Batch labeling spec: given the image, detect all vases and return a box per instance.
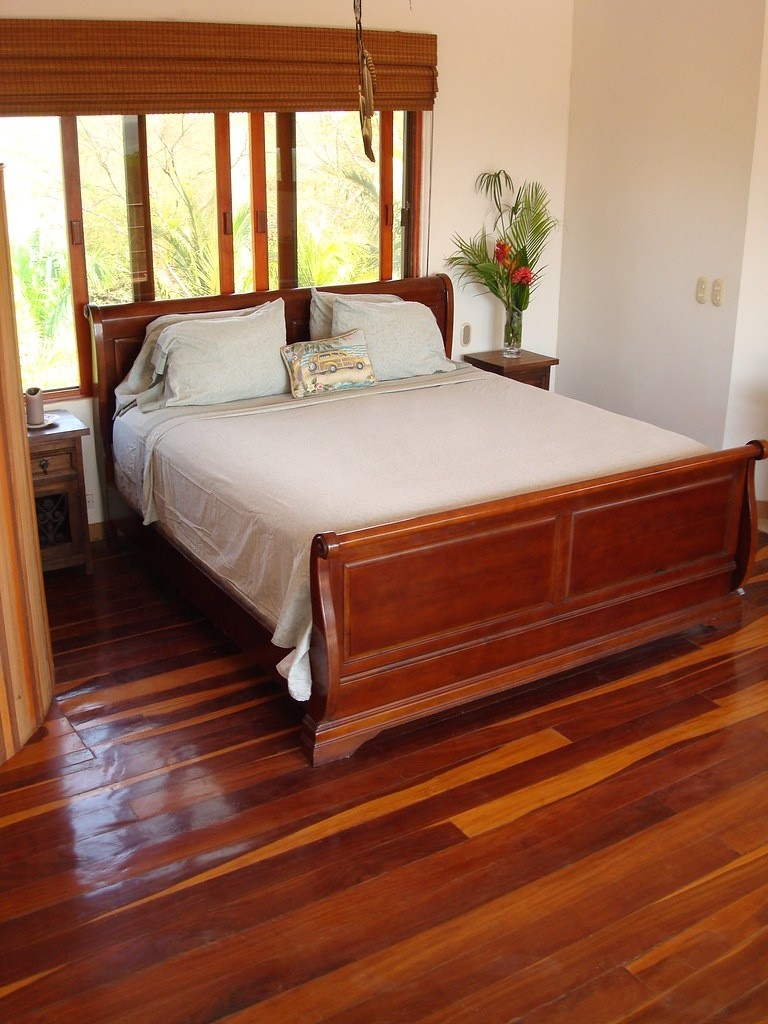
[502,304,525,359]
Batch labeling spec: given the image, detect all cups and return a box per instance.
[26,387,44,424]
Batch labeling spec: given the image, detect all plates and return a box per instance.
[25,413,60,429]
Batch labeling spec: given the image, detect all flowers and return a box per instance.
[443,167,561,347]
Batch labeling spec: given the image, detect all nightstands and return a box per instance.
[25,406,92,576]
[461,346,559,391]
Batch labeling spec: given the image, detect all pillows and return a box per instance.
[330,297,471,380]
[280,327,377,401]
[147,296,292,409]
[309,287,404,339]
[113,302,269,410]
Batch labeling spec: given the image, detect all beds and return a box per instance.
[83,273,767,772]
